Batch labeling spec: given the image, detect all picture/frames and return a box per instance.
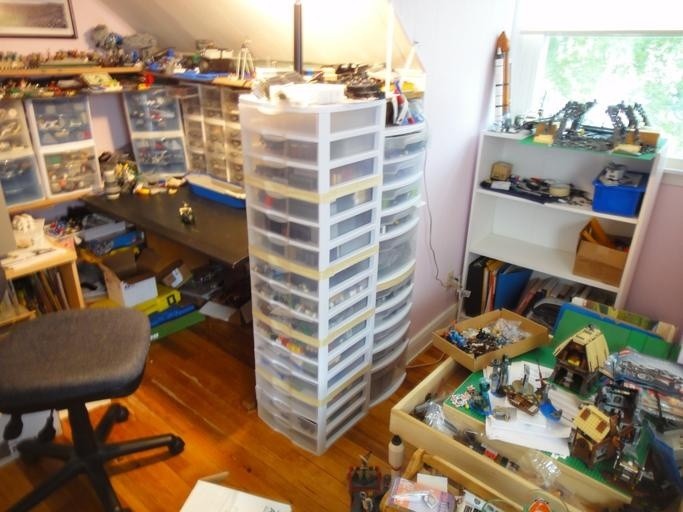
[0,0,78,39]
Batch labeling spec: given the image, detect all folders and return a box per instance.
[494,264,533,309]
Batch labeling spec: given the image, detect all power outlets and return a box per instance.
[451,273,461,286]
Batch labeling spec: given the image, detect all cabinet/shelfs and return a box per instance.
[24,93,104,200]
[176,80,245,190]
[0,97,46,207]
[122,83,189,178]
[455,124,671,322]
[0,225,86,308]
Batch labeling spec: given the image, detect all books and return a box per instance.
[7,267,71,315]
[513,277,612,318]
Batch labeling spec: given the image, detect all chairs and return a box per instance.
[0,264,184,511]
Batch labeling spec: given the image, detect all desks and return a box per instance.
[79,183,249,272]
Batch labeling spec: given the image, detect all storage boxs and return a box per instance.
[0,310,64,466]
[197,289,253,328]
[95,246,159,308]
[591,168,649,218]
[205,317,254,369]
[159,258,194,289]
[179,270,227,310]
[572,218,632,288]
